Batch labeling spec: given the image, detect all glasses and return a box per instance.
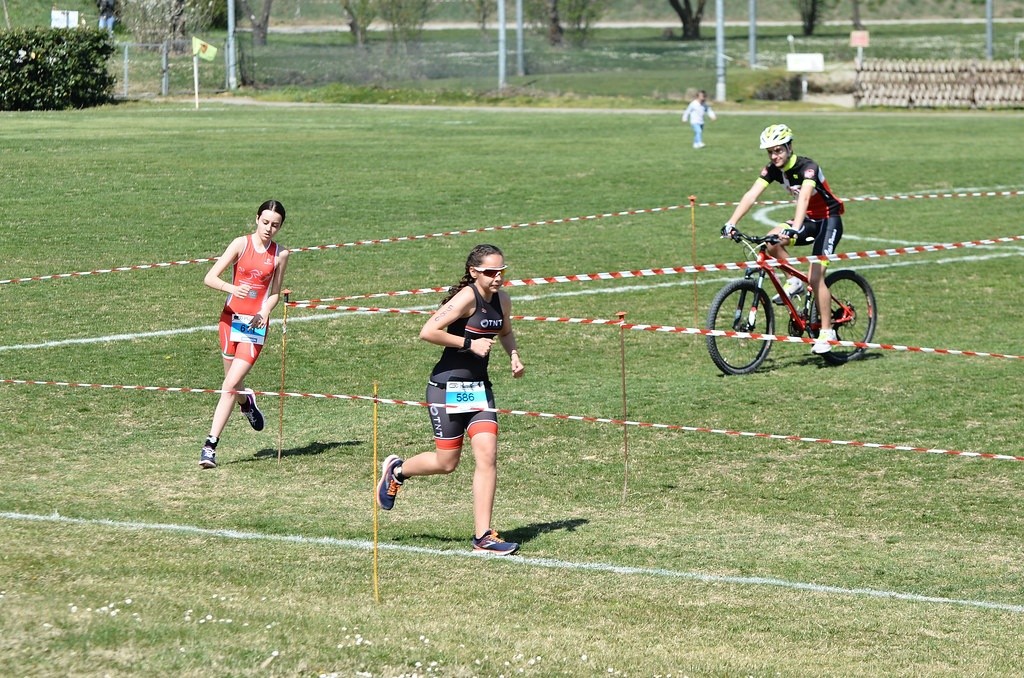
[473,265,508,278]
[768,146,786,153]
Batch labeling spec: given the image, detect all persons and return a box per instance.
[96,0,118,38]
[721,124,844,354]
[198,200,290,468]
[377,244,524,555]
[681,90,717,148]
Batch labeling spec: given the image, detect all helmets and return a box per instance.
[759,124,792,149]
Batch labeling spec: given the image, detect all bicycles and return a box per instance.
[702,229,881,373]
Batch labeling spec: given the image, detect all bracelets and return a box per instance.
[510,352,520,360]
[458,338,471,352]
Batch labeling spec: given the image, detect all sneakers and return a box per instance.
[240,388,264,431]
[811,329,838,353]
[377,455,404,510]
[772,278,804,305]
[199,446,216,467]
[473,529,520,556]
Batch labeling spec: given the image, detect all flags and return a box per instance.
[193,37,218,61]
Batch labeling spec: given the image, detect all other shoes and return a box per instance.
[693,142,705,149]
